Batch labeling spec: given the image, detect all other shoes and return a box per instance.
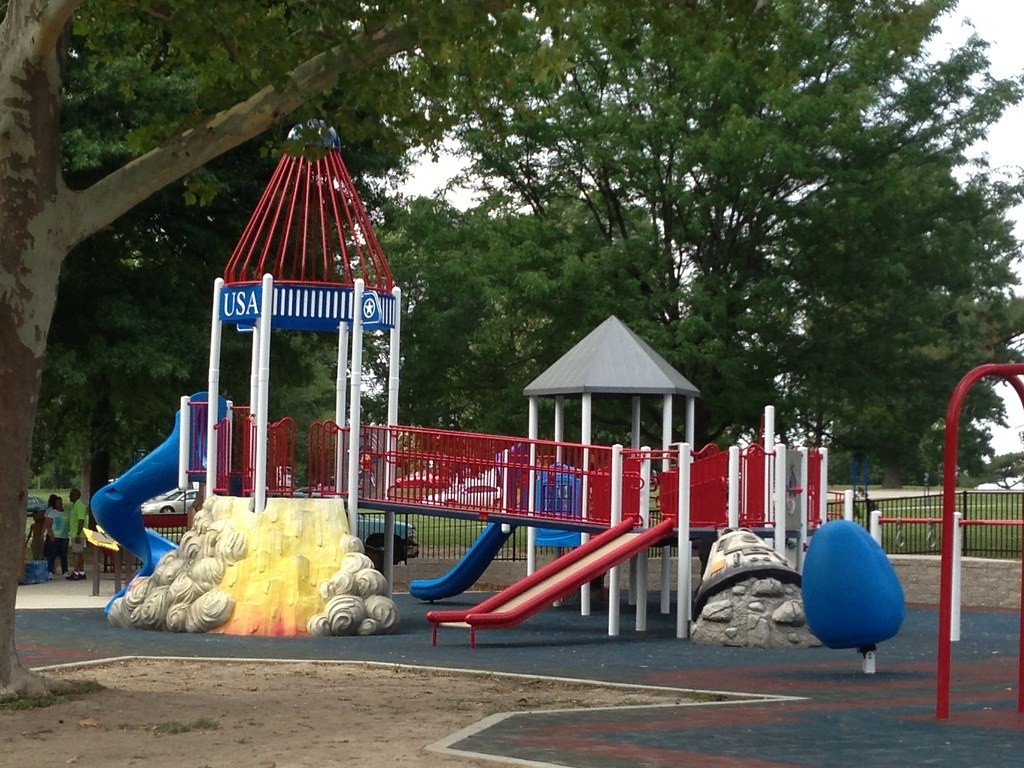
[49,572,54,580]
[63,572,70,577]
[65,572,86,581]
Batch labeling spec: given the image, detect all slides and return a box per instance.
[408,522,518,600]
[426,516,674,630]
[90,392,228,615]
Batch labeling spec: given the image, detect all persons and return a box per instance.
[923,473,932,494]
[25,494,70,580]
[65,489,87,581]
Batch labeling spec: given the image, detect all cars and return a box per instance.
[141,490,200,514]
[345,509,420,573]
